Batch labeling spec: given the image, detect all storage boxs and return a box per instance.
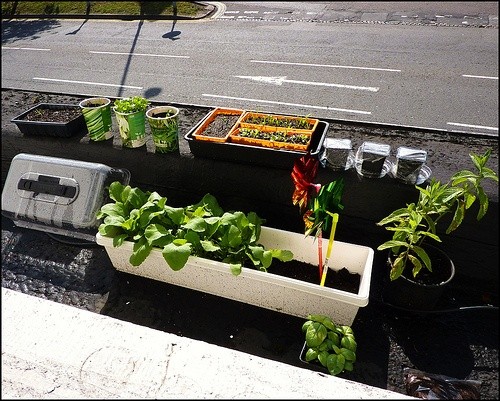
[1,152,132,242]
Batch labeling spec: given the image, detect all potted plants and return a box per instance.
[238,111,319,133]
[192,105,247,141]
[299,313,357,379]
[375,147,499,318]
[146,105,181,155]
[10,102,88,138]
[95,179,375,327]
[79,95,114,141]
[113,94,151,147]
[229,125,312,151]
